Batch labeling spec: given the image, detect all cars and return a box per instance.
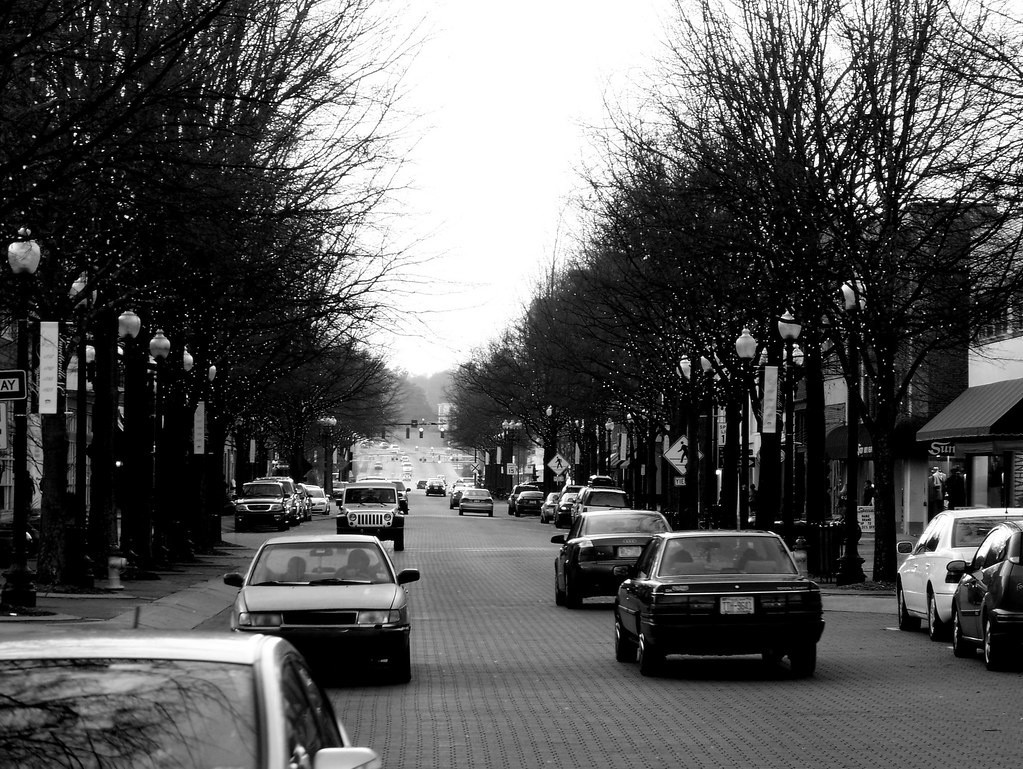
[946,520,1023,673]
[0,629,383,769]
[222,534,420,683]
[550,509,675,608]
[611,528,824,679]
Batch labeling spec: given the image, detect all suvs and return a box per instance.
[897,505,1023,643]
[228,437,630,552]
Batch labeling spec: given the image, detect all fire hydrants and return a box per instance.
[105,547,129,592]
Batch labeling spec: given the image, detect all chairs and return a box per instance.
[669,562,707,575]
[745,560,779,574]
[279,556,310,581]
[333,550,377,580]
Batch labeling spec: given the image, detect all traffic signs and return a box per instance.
[1,368,29,403]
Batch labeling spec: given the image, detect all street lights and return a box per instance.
[115,306,140,560]
[148,326,172,566]
[732,326,758,530]
[674,352,698,530]
[68,267,98,569]
[699,346,720,530]
[776,304,802,552]
[833,268,866,585]
[605,417,614,475]
[0,227,38,608]
[626,411,637,509]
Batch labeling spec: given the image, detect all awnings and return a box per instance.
[605,453,636,469]
[916,379,1023,440]
[826,420,911,458]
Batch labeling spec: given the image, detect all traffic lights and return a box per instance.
[440,427,444,438]
[419,427,424,438]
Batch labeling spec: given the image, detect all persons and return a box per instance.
[749,484,758,515]
[929,467,966,510]
[861,480,873,525]
[835,484,847,517]
[669,549,693,570]
[738,548,758,570]
[342,550,375,581]
[288,557,306,582]
